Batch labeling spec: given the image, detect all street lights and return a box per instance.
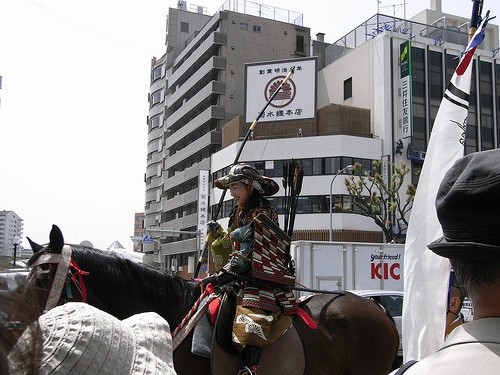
[329,164,353,241]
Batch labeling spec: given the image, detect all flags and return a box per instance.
[398,17,490,367]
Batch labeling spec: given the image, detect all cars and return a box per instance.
[346,287,416,363]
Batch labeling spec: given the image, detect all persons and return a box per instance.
[197,163,280,374]
[385,147,500,375]
[7,300,179,375]
[442,271,468,343]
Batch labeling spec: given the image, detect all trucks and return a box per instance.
[290,238,407,305]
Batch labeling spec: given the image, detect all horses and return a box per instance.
[10,223,401,375]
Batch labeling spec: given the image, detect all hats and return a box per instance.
[427,148,500,261]
[7,301,177,375]
[214,163,279,196]
